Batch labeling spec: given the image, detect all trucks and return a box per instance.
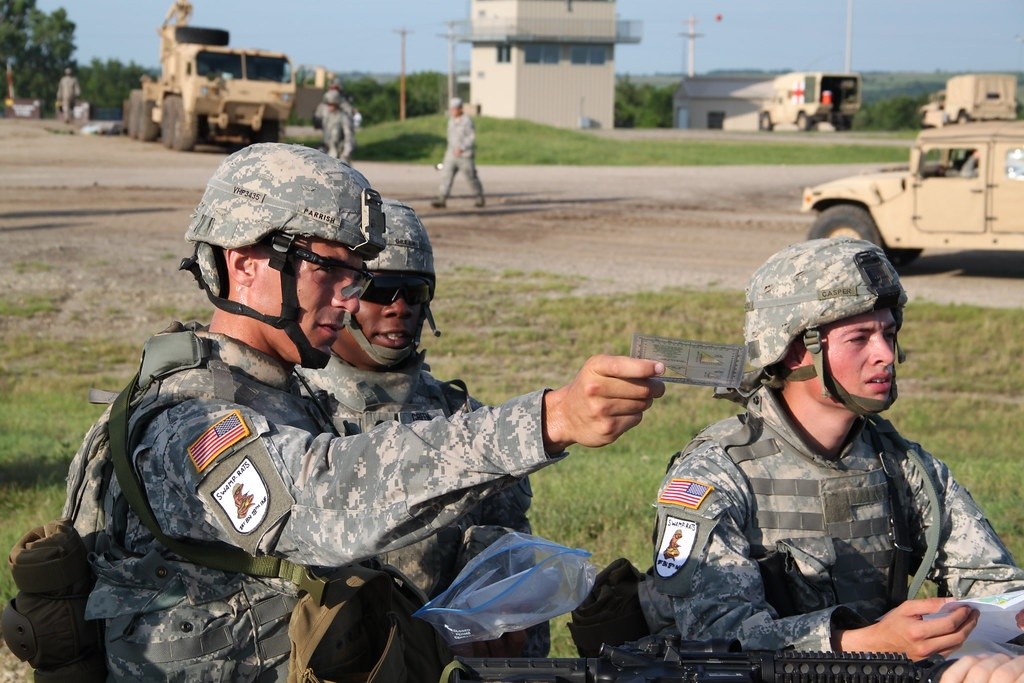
[920,73,1017,129]
[758,72,861,130]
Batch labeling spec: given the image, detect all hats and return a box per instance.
[450,97,462,107]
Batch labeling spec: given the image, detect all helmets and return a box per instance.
[183,141,387,298]
[742,233,908,369]
[362,199,435,278]
[321,89,341,105]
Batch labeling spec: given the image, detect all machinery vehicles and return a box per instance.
[293,66,332,127]
[122,1,296,152]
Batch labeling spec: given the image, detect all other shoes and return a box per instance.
[474,197,485,207]
[430,201,447,208]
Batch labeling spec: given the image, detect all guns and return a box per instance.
[449,632,960,683]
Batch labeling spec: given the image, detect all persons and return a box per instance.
[322,90,354,162]
[64,142,666,683]
[432,98,484,206]
[293,198,550,660]
[938,149,979,177]
[57,68,81,123]
[654,237,1023,683]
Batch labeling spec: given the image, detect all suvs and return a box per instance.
[798,120,1024,266]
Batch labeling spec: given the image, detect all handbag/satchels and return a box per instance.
[286,564,468,683]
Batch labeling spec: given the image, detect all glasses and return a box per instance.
[356,273,434,306]
